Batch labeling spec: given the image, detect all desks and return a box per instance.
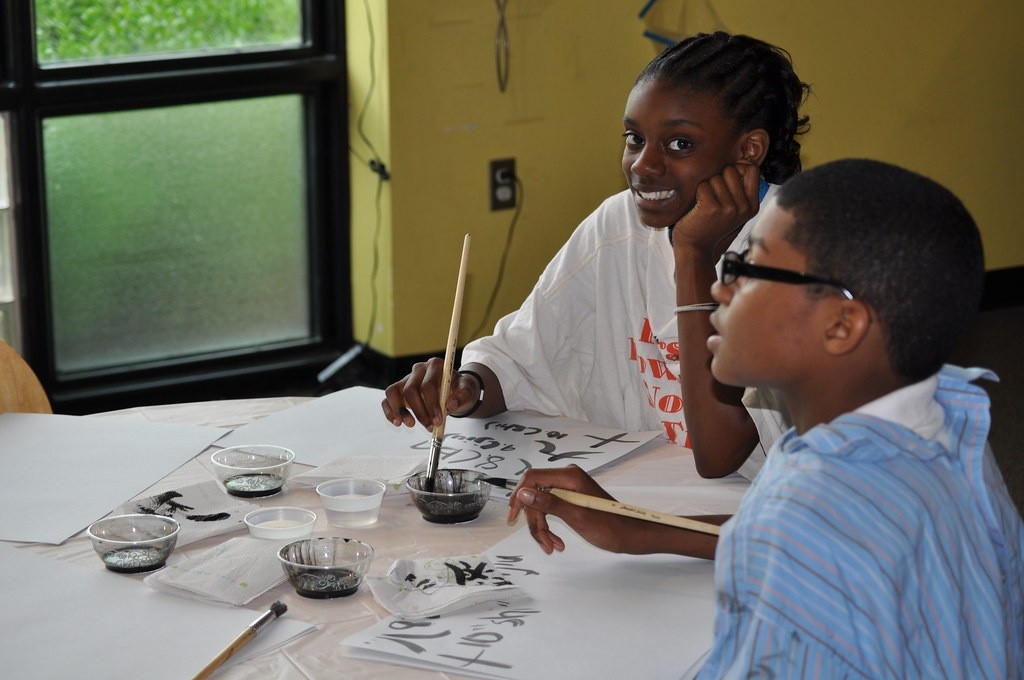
[0,396,751,680]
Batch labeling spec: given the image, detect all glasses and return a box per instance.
[716,251,876,324]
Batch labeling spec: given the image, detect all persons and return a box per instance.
[506,157,1023,680]
[380,31,812,480]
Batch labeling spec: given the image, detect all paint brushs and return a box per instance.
[423,234,473,493]
[503,476,721,541]
[191,601,288,680]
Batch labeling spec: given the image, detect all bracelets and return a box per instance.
[449,370,485,418]
[673,302,720,314]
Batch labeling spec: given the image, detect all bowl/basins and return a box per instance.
[210,444,296,498]
[86,514,182,574]
[315,477,387,528]
[405,468,493,526]
[276,536,375,599]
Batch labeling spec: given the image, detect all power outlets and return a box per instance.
[489,158,512,213]
